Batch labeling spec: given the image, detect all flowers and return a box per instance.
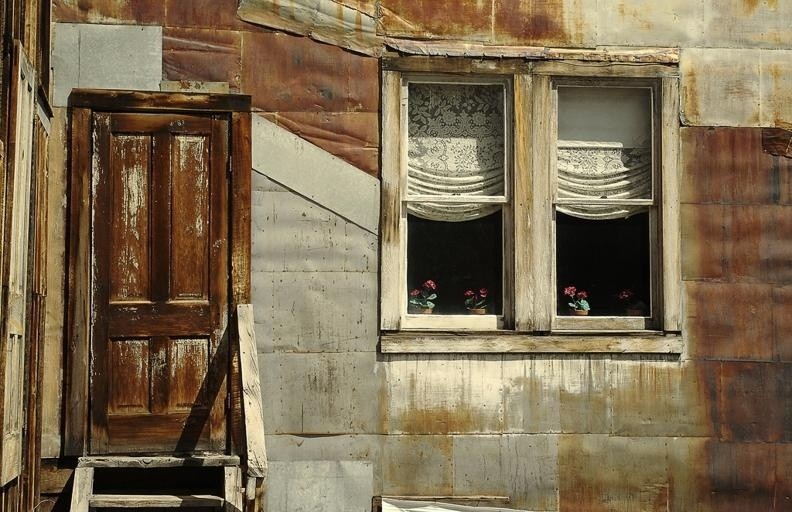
[463,287,489,309]
[563,283,591,311]
[408,279,437,309]
[615,287,649,310]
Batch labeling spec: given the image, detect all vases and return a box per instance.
[468,307,486,315]
[569,309,586,316]
[626,308,643,316]
[414,306,433,314]
